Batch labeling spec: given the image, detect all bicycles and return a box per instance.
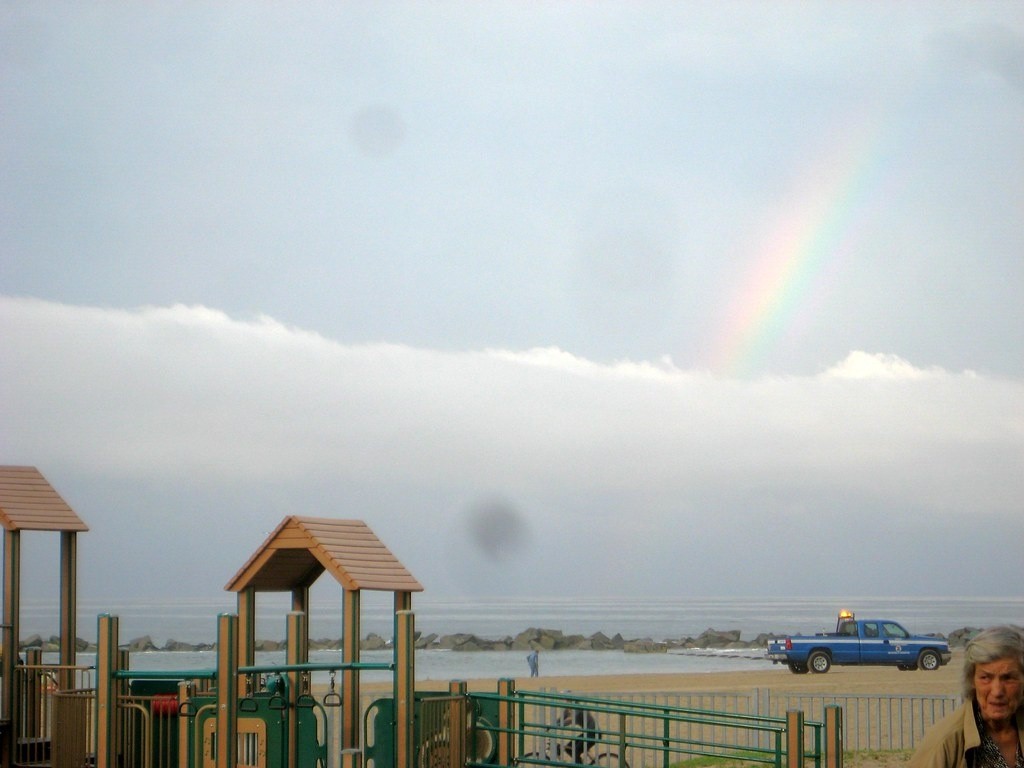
[516,728,630,768]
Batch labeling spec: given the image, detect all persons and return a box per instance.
[544,689,602,765]
[906,627,1024,768]
[526,650,539,678]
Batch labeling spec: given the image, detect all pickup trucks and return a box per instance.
[763,609,952,675]
